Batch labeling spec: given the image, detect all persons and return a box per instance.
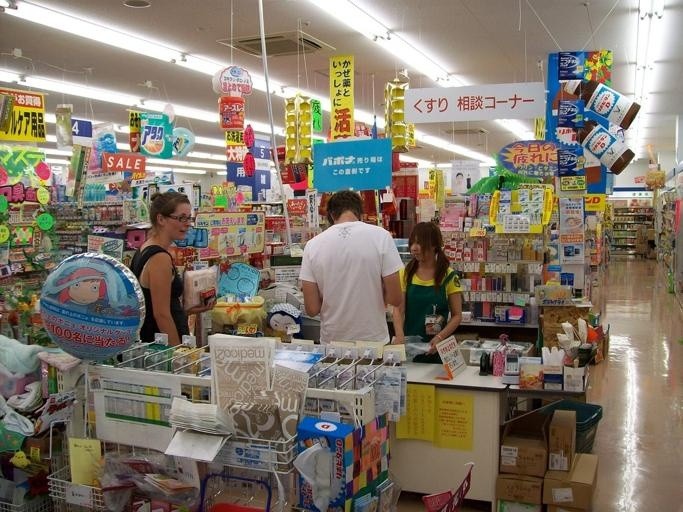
[129,190,196,348]
[391,220,465,363]
[453,173,467,194]
[296,190,405,365]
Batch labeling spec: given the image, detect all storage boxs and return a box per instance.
[494,410,599,512]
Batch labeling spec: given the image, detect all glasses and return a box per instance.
[169,215,192,223]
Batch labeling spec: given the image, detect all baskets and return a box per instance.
[545,400,602,453]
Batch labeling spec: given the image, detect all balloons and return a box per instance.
[38,252,146,364]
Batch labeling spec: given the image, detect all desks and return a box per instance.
[386,361,590,512]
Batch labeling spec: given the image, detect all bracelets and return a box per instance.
[436,334,444,341]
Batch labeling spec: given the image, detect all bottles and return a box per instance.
[81,182,122,222]
